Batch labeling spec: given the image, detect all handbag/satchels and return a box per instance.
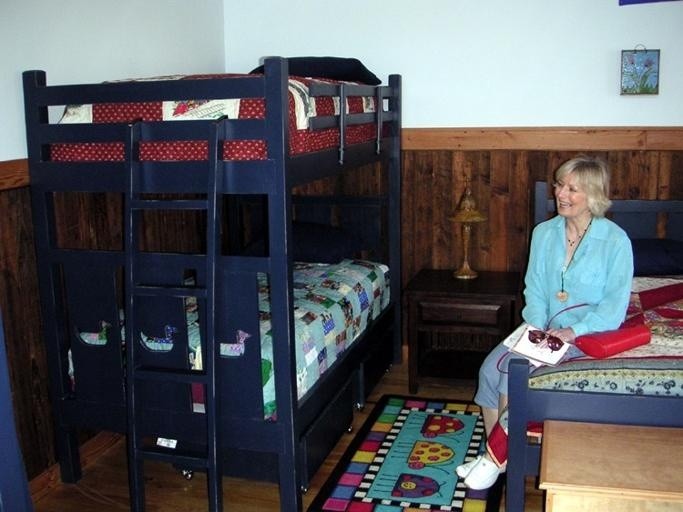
[571,323,652,360]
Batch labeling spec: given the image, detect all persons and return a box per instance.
[452,152,634,492]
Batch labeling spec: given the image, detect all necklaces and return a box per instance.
[563,222,590,246]
[556,215,593,303]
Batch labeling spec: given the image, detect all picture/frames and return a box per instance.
[620,49,660,95]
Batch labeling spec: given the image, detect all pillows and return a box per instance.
[250,56,381,85]
[631,239,683,276]
[242,221,354,264]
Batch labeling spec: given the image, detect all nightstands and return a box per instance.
[408,269,524,395]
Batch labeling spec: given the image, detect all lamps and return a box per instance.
[451,186,489,283]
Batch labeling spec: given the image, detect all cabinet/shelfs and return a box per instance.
[539,419,683,512]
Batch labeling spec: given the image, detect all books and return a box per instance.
[503,322,572,369]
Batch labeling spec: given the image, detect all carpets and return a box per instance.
[306,393,504,512]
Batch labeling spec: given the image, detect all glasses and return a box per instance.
[528,329,565,352]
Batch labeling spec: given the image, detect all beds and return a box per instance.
[508,180,683,512]
[21,57,404,508]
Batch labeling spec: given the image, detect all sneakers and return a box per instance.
[455,453,507,480]
[462,454,501,492]
